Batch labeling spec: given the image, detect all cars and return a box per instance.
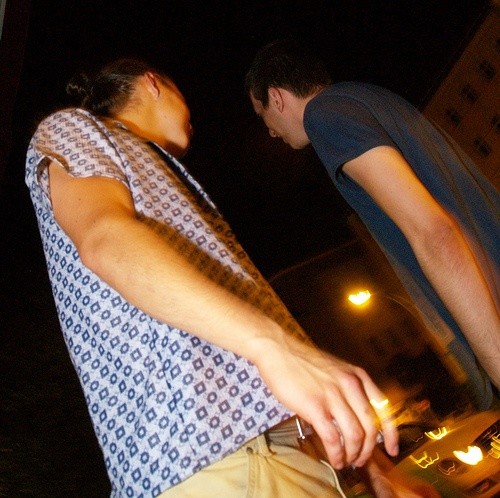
[340,410,499,498]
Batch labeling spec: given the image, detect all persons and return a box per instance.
[25,58,442,498]
[247,55,500,408]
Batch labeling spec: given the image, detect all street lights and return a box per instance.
[349,290,462,376]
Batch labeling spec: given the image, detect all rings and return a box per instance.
[373,398,391,409]
